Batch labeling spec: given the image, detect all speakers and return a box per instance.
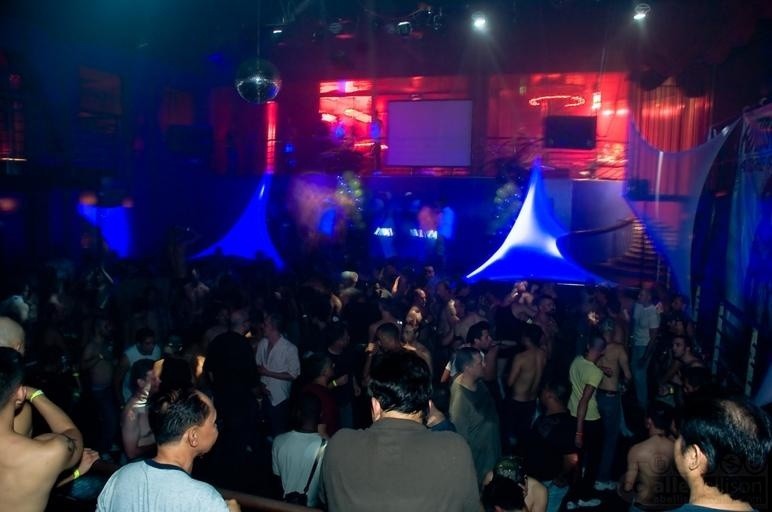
[544,116,596,149]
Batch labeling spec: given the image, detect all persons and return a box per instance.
[319,347,481,512]
[673,385,770,512]
[374,266,711,511]
[2,261,159,511]
[158,262,382,511]
[97,380,240,511]
[1,347,83,511]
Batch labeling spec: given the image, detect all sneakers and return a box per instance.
[566,496,604,511]
[592,479,619,493]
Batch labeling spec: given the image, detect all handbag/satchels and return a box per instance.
[283,437,327,508]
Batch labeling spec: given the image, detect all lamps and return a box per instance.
[232,0,282,106]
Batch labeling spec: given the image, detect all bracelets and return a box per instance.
[30,390,44,402]
[72,466,79,479]
